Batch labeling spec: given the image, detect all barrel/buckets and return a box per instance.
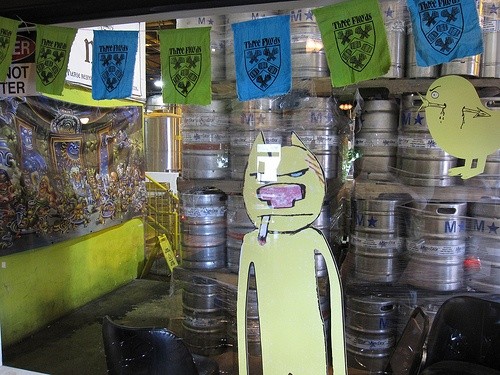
[179,0,500,372]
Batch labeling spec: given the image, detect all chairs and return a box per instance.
[421,297,500,375]
[378,305,430,375]
[102,316,221,375]
